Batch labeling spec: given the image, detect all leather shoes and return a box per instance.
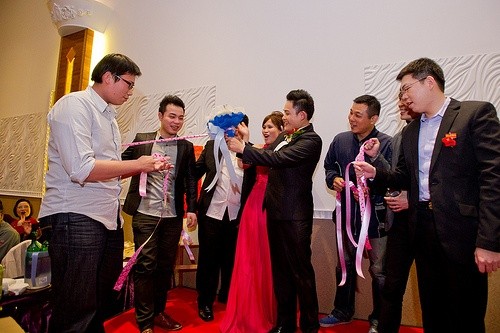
[198,304,214,321]
[141,311,183,333]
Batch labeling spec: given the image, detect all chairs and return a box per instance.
[1,240,45,280]
[175,218,200,286]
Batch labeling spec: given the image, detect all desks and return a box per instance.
[0,257,131,333]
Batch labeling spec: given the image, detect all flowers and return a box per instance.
[442,132,457,148]
[284,127,306,144]
[204,102,249,195]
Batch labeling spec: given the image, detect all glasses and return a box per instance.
[112,73,135,90]
[400,76,428,94]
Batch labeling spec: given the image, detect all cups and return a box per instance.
[0,262,3,296]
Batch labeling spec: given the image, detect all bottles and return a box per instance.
[26,232,41,278]
[385,187,401,197]
[40,241,51,274]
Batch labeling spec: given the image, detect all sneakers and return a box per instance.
[369,318,379,332]
[320,313,354,327]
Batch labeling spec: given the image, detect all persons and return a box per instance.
[319,96,391,328]
[122,95,198,333]
[195,89,320,333]
[396,58,500,333]
[10,200,42,238]
[0,200,21,265]
[38,53,175,333]
[370,87,422,333]
